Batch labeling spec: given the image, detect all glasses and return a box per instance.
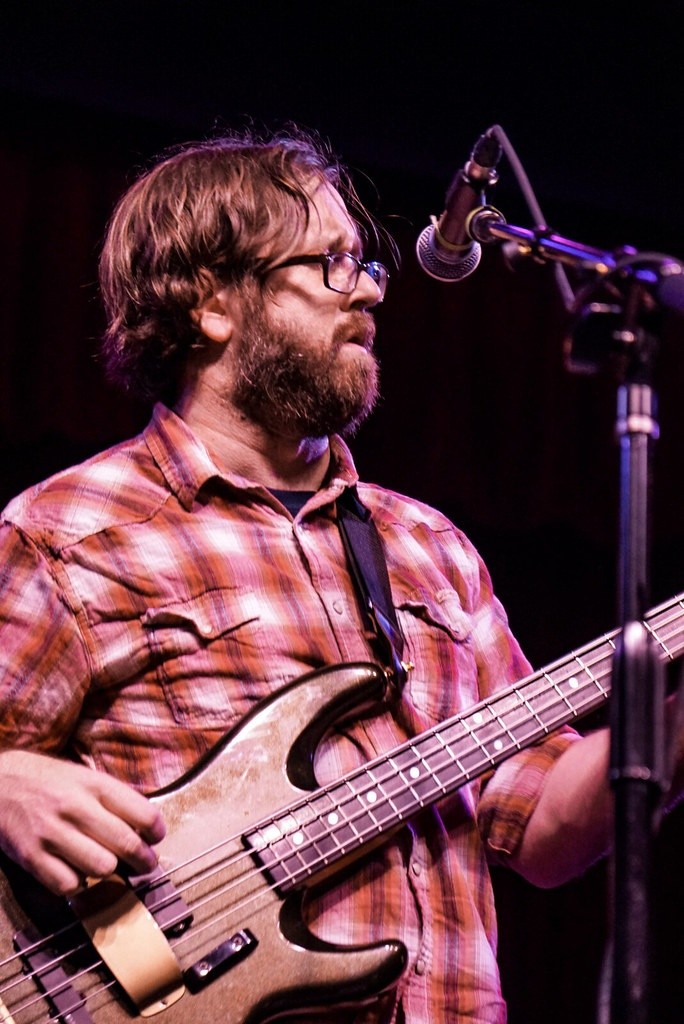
[258,252,391,304]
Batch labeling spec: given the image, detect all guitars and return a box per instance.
[1,589,684,1024]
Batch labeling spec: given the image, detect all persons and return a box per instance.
[0,140,684,1024]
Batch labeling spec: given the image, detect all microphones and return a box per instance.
[417,132,504,282]
